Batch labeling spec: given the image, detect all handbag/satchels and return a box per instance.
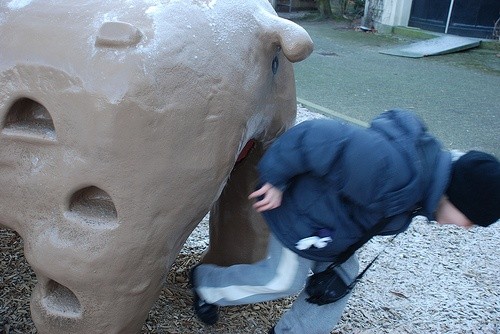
[304,266,351,308]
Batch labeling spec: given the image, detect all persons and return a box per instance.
[189,109,500,334]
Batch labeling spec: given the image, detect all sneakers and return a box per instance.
[188,263,220,326]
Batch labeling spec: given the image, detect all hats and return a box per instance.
[446,146,500,228]
